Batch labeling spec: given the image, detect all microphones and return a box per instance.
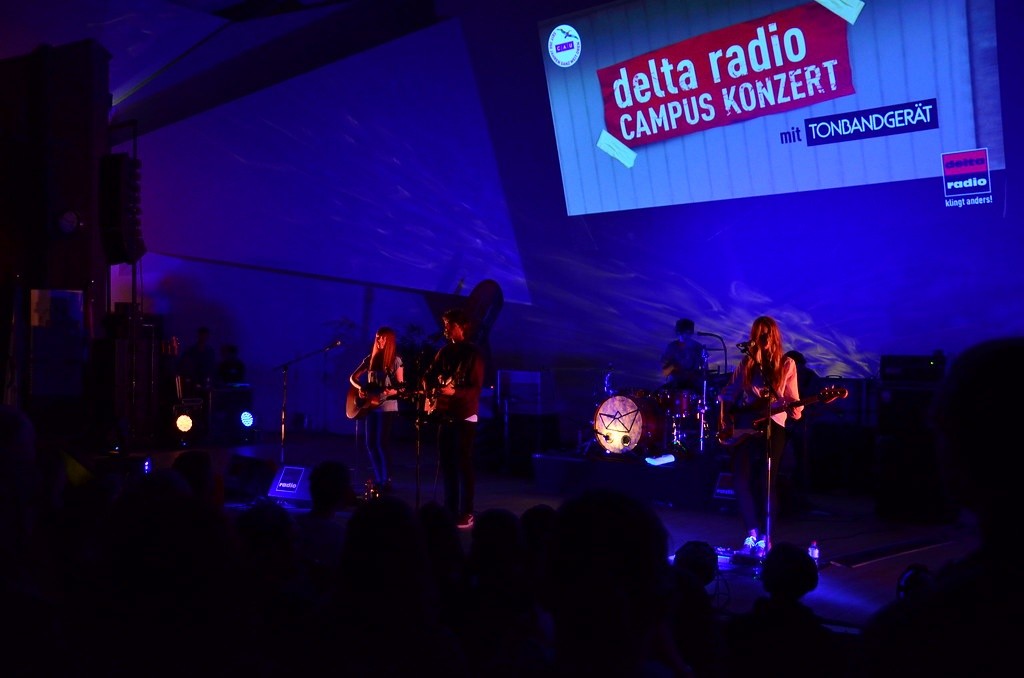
[697,332,711,336]
[325,340,342,352]
[737,340,755,347]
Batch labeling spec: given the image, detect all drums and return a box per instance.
[590,373,732,455]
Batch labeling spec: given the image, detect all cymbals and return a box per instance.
[697,369,718,374]
[693,347,724,351]
[594,368,627,374]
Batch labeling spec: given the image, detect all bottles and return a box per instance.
[808,540,819,569]
[364,479,374,499]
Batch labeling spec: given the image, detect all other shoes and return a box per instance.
[755,534,772,557]
[455,512,475,529]
[739,535,757,555]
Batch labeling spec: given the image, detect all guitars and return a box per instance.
[346,362,464,421]
[716,383,849,447]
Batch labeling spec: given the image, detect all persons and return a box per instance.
[0,340,1024,678]
[85,312,134,433]
[421,308,485,528]
[718,316,805,556]
[347,328,404,486]
[176,326,245,438]
[662,318,709,385]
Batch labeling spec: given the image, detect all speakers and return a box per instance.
[99,151,148,266]
[268,464,314,510]
[97,454,153,501]
[482,411,560,476]
[809,423,878,497]
